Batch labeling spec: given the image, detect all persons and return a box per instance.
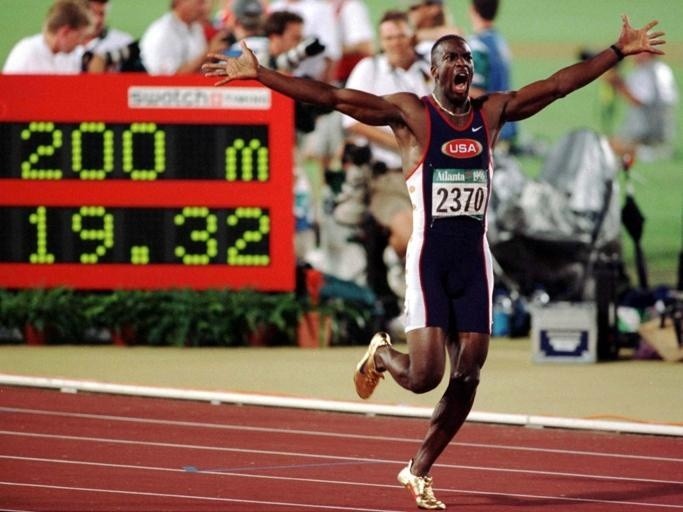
[272,2,513,315]
[606,36,666,161]
[201,13,667,511]
[6,1,301,76]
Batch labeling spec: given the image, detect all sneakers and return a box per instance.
[396,458,446,510]
[353,332,392,399]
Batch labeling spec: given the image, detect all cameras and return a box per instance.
[81,40,144,73]
[269,37,325,71]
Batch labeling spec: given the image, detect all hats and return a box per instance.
[230,0,262,26]
